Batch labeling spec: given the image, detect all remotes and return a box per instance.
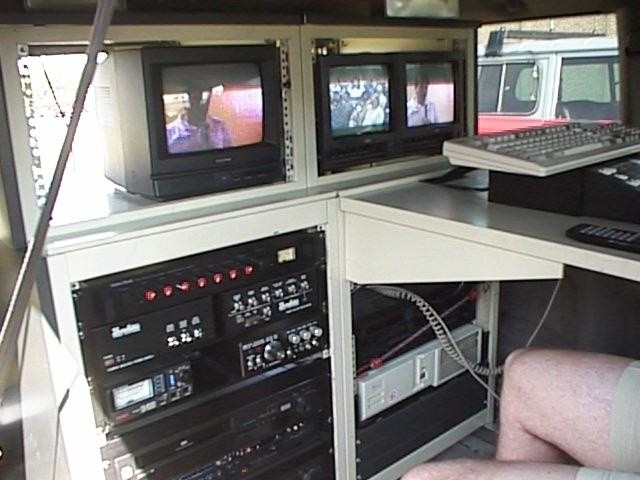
[565,223,639,253]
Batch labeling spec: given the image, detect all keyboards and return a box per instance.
[443,121,639,177]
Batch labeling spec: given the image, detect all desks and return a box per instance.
[327,167,640,480]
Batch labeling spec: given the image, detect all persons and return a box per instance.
[399,347,640,480]
[331,74,389,133]
[165,86,235,154]
[407,72,439,130]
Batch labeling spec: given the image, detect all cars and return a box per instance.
[477,26,619,133]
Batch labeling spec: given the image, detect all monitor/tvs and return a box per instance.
[312,52,467,177]
[94,41,286,203]
